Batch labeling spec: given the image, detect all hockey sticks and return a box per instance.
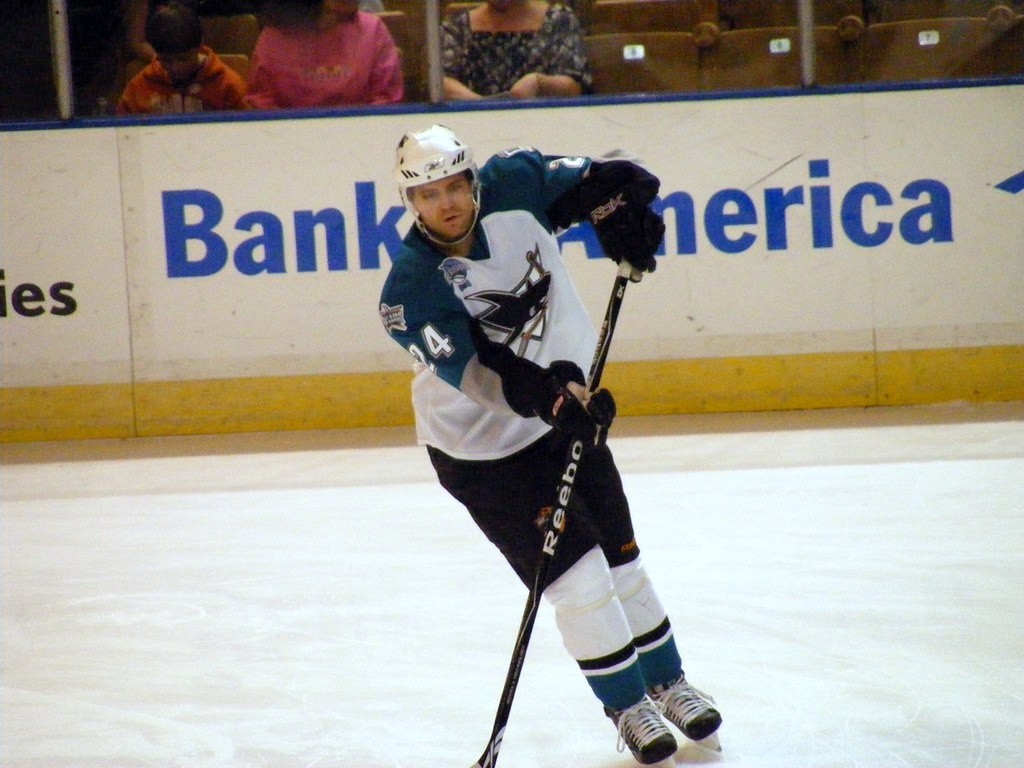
[472,253,636,768]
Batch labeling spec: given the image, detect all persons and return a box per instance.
[377,124,723,768]
[110,0,248,116]
[242,0,406,110]
[440,0,592,100]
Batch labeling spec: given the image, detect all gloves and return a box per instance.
[532,360,616,446]
[586,171,665,272]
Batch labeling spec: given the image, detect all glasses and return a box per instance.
[155,46,196,63]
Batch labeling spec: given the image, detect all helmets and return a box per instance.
[392,127,481,217]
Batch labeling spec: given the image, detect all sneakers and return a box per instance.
[647,671,723,752]
[604,694,678,768]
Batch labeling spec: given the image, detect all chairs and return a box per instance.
[124,0,1024,106]
